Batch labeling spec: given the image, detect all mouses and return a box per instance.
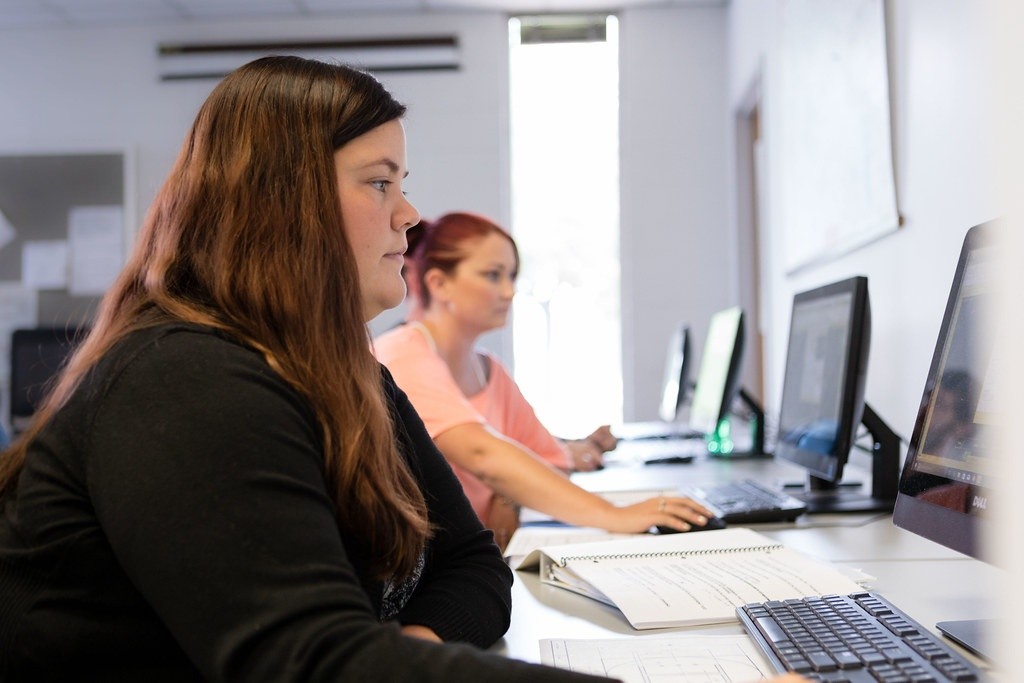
[657,516,726,535]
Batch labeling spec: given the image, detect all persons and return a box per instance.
[363,219,617,472]
[369,214,714,550]
[0,56,815,683]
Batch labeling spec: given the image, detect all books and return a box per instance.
[513,528,875,630]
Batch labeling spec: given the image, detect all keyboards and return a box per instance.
[683,477,807,525]
[735,591,1003,683]
[643,455,694,464]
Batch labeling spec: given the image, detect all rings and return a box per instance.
[660,501,665,513]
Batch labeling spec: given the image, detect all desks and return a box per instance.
[476,418,1000,683]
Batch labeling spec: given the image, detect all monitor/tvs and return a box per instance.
[657,328,706,441]
[892,211,1017,662]
[688,307,774,460]
[13,327,91,432]
[773,276,903,515]
